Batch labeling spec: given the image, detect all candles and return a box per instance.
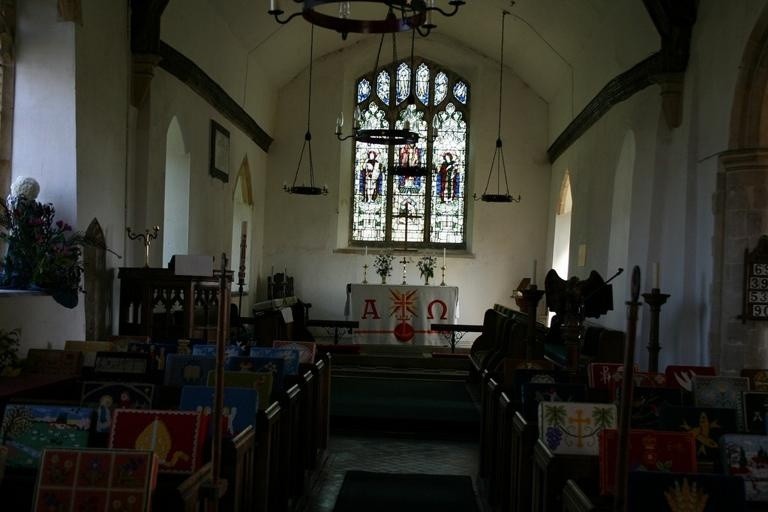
[364,244,367,267]
[649,257,660,289]
[282,263,287,281]
[269,263,274,283]
[442,246,446,267]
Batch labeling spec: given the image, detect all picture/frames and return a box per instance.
[206,118,235,184]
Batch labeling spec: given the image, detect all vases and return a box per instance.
[423,271,429,285]
[380,272,386,285]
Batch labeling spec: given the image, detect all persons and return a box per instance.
[358,151,386,202]
[438,153,460,203]
[399,143,422,188]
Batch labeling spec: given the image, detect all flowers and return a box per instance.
[414,254,438,279]
[0,171,87,311]
[372,243,396,276]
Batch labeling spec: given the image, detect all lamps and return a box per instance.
[281,18,333,197]
[471,9,524,203]
[334,4,439,147]
[264,0,465,39]
[381,141,438,181]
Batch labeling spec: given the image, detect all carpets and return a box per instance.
[329,468,478,511]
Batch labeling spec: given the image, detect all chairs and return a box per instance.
[478,365,766,512]
[0,333,333,512]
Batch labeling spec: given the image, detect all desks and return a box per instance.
[342,282,458,354]
